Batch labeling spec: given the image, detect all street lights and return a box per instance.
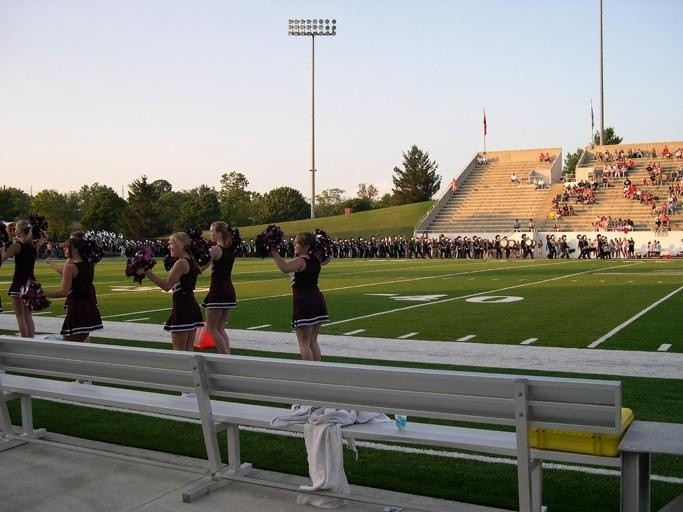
[286,17,335,218]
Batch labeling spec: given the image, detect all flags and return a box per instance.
[483,113,486,136]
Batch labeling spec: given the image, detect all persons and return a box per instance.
[37,238,104,342]
[134,231,204,352]
[415,145,683,261]
[190,220,237,354]
[269,231,332,361]
[0,220,38,339]
[39,228,415,260]
[45,231,99,342]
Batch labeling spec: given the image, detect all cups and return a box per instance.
[393,414,408,432]
